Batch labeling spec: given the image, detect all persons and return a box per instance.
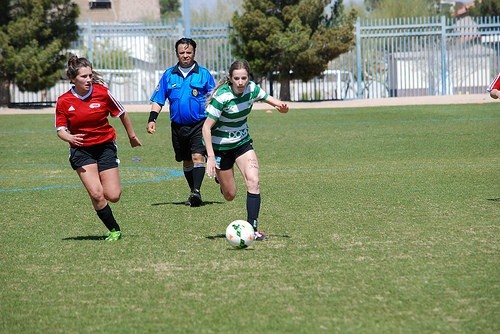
[146,38,216,207]
[54,56,142,241]
[486,73,500,99]
[203,59,289,240]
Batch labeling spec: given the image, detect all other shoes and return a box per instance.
[185,188,204,207]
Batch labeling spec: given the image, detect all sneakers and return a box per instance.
[105,228,121,242]
[253,231,268,241]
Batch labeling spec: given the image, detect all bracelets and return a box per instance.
[148,111,159,124]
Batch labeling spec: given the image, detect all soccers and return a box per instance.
[226,220,256,248]
